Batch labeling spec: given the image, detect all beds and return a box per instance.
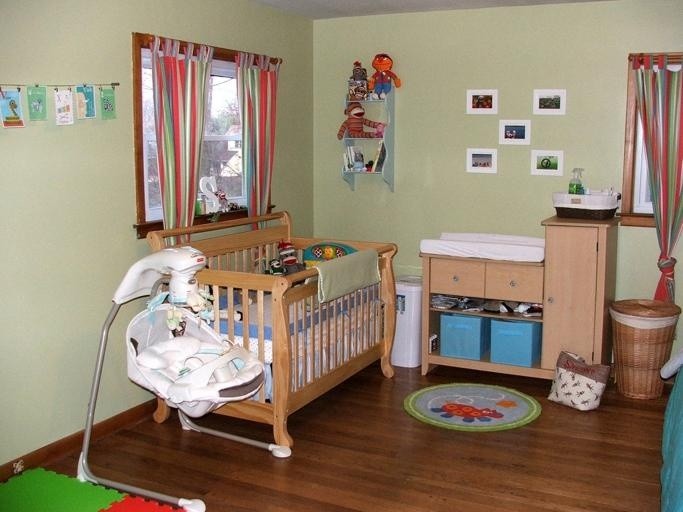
[145,211,400,454]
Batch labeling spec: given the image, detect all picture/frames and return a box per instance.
[465,87,567,178]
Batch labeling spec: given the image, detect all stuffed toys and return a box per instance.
[277,241,306,287]
[345,60,369,101]
[263,257,287,276]
[336,100,386,142]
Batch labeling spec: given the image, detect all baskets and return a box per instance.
[609,299,682,400]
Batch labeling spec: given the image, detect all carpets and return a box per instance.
[403,381,543,434]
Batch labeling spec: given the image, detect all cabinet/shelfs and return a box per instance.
[340,84,395,191]
[418,213,618,387]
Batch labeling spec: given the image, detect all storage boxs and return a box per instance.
[440,312,542,368]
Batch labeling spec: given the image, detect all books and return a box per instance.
[342,140,387,173]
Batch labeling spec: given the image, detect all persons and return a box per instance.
[366,52,402,100]
